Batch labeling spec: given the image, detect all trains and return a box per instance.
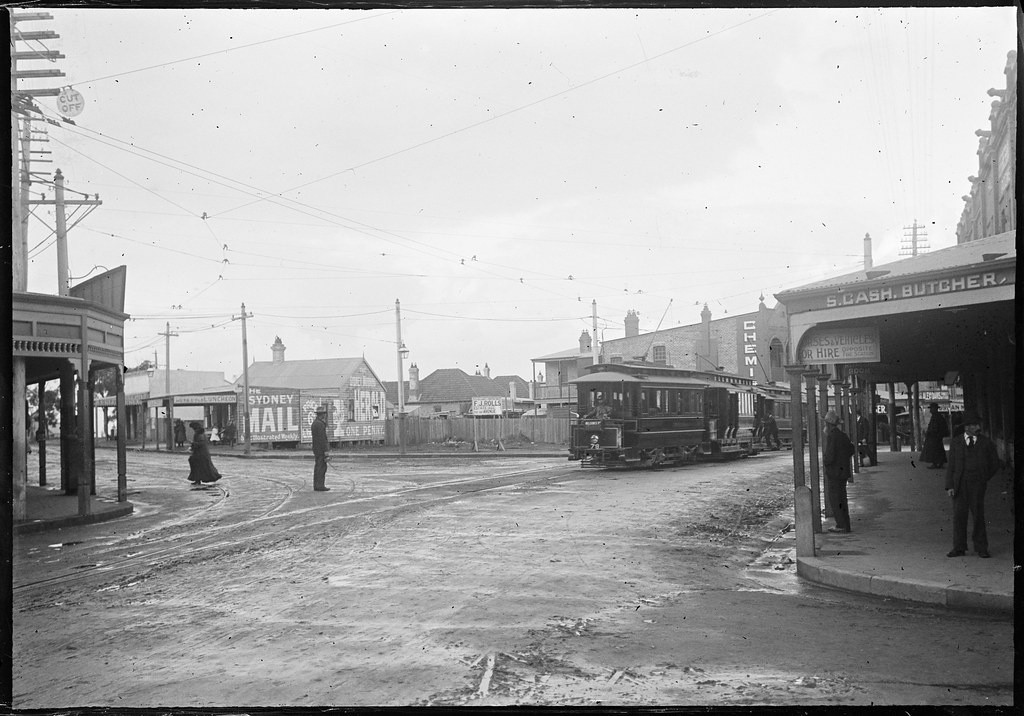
[567,355,887,470]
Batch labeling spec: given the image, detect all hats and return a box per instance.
[959,411,983,425]
[314,407,327,413]
[825,411,843,425]
[928,403,940,408]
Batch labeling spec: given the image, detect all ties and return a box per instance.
[968,436,974,446]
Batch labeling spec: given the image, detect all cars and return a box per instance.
[894,409,967,450]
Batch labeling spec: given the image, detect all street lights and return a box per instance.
[394,299,410,413]
[144,322,179,395]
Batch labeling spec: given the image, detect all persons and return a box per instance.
[583,395,613,420]
[187,421,222,486]
[945,411,999,558]
[918,403,948,470]
[310,407,330,492]
[821,410,855,533]
[173,418,186,448]
[209,422,221,447]
[856,410,877,467]
[763,413,783,451]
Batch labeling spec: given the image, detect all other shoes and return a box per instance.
[927,463,943,468]
[979,552,990,558]
[314,487,330,491]
[828,526,851,533]
[191,481,201,484]
[947,550,964,557]
